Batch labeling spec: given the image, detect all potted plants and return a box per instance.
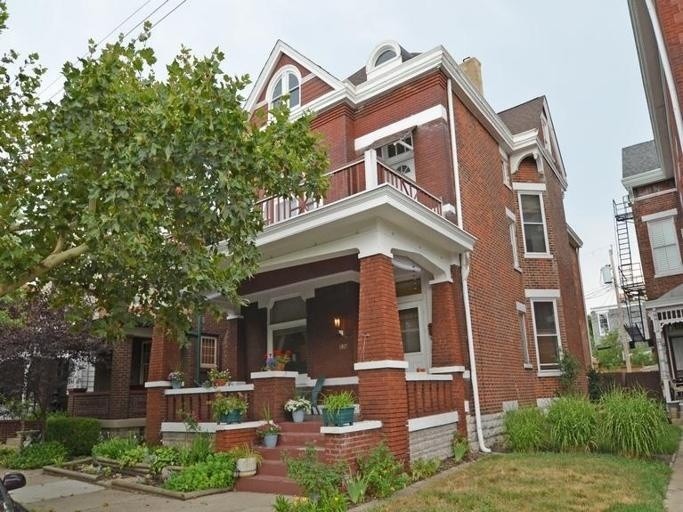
[207,367,232,387]
[168,369,185,389]
[256,405,283,448]
[230,444,263,478]
[208,395,248,423]
[320,391,355,426]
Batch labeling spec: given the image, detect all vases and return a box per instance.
[292,411,305,423]
[270,363,286,371]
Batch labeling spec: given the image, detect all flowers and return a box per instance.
[263,345,291,364]
[284,393,318,419]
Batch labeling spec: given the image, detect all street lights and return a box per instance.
[189,293,209,387]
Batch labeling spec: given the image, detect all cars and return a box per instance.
[0,472,29,512]
